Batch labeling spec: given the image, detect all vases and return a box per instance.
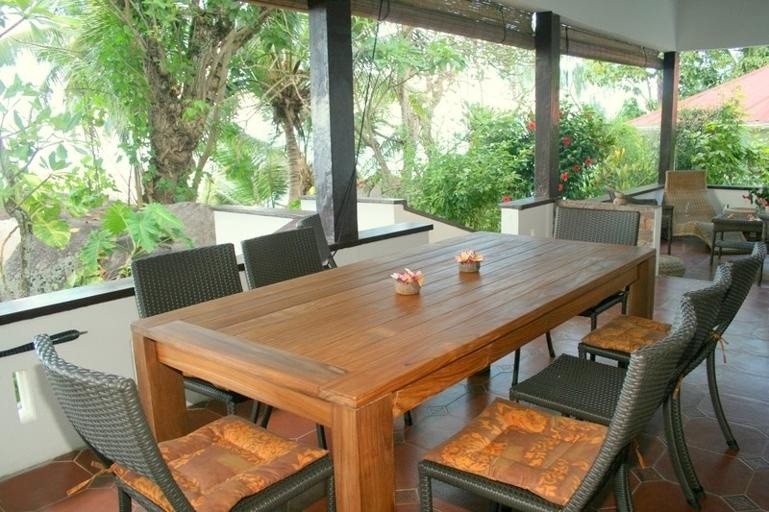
[458,261,481,273]
[393,278,420,296]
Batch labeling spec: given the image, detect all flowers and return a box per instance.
[456,246,484,265]
[391,266,424,288]
[740,181,769,212]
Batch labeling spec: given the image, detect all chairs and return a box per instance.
[130,243,253,416]
[416,294,698,512]
[663,169,749,254]
[512,205,643,385]
[573,239,768,501]
[241,226,413,450]
[489,263,733,511]
[32,333,335,512]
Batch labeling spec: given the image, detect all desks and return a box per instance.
[710,203,763,267]
[130,231,656,512]
